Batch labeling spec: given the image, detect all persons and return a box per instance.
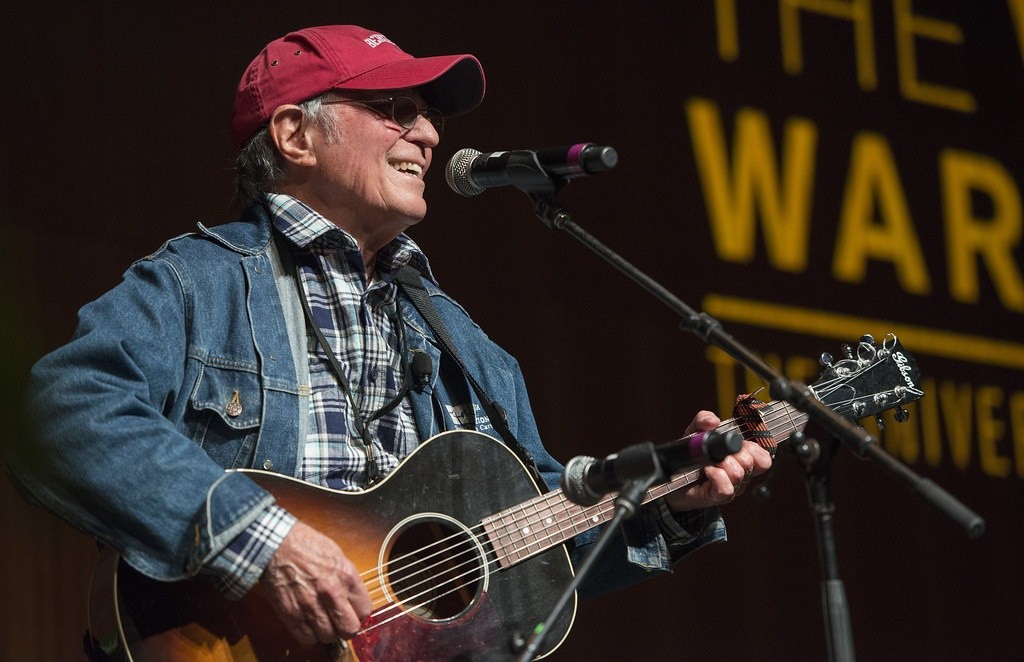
[9,25,773,646]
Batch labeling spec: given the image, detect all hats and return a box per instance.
[228,25,486,151]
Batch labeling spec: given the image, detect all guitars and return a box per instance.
[83,325,925,662]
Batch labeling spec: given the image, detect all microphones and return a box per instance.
[445,142,618,197]
[561,431,743,507]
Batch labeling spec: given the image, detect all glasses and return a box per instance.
[309,96,445,138]
